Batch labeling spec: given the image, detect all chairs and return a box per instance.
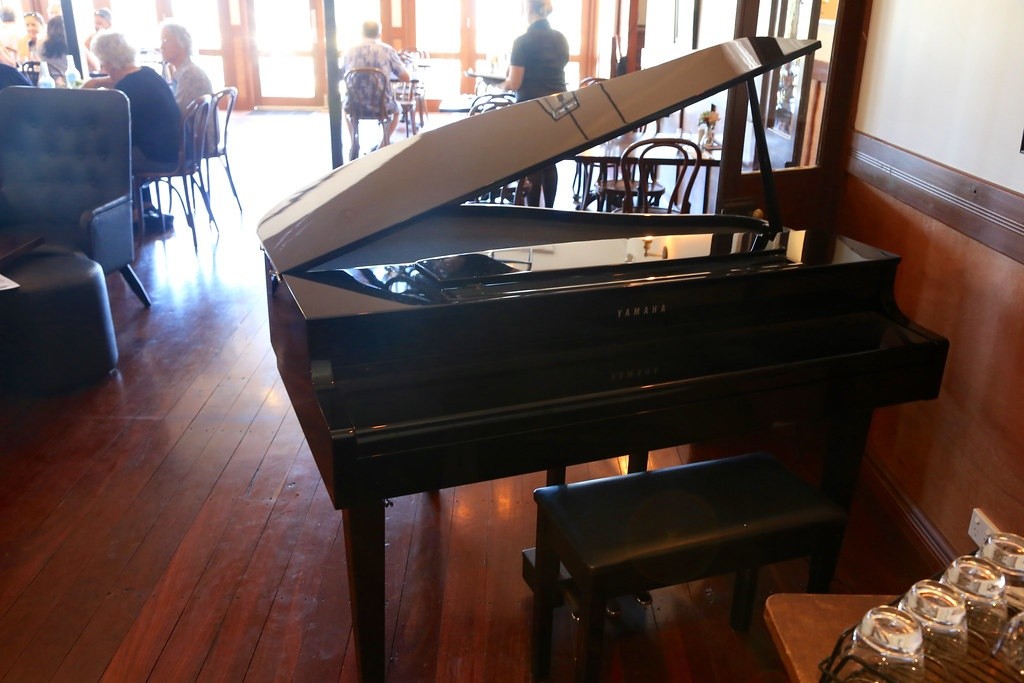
[397,45,430,136]
[168,86,243,230]
[343,67,392,162]
[22,61,40,86]
[0,84,154,310]
[133,93,220,258]
[468,77,703,214]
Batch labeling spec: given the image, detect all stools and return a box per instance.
[0,253,120,397]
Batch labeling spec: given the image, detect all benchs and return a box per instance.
[529,450,849,683]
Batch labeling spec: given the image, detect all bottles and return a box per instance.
[38,63,55,89]
[65,55,80,88]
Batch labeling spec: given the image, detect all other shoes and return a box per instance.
[345,145,360,161]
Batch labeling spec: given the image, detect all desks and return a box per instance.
[389,73,419,137]
[571,130,722,214]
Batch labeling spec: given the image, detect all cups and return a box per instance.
[974,532,1024,614]
[898,580,968,665]
[936,554,1007,661]
[842,604,926,683]
[993,612,1024,678]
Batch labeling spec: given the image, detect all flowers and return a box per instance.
[698,110,721,129]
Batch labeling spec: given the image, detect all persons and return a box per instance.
[339,20,410,162]
[0,5,27,51]
[38,15,100,87]
[0,40,34,89]
[80,30,183,234]
[161,22,221,156]
[482,1,570,208]
[83,7,112,78]
[16,11,47,67]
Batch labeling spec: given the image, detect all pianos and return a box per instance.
[252,31,955,682]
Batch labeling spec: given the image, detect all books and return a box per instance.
[463,67,508,81]
